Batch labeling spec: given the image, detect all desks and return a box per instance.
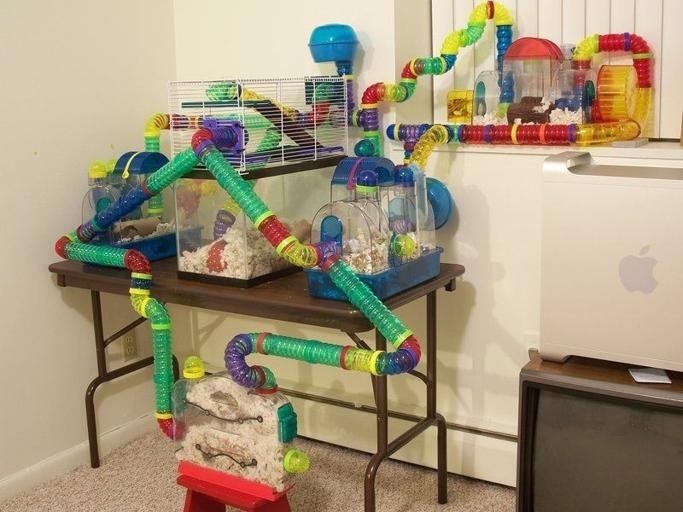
[49,238,466,511]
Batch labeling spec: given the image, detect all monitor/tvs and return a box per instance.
[513,346,683,512]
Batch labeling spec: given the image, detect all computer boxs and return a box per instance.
[536,145,683,373]
